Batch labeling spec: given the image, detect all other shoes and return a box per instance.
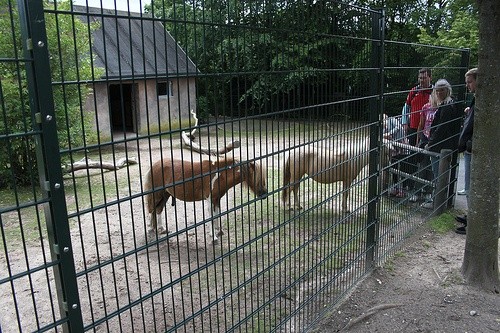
[409,195,418,202]
[396,191,409,197]
[455,215,467,226]
[390,188,397,195]
[454,226,467,234]
[421,200,434,209]
[457,190,466,195]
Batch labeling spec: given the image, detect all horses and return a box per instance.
[282,127,393,213]
[144,158,270,243]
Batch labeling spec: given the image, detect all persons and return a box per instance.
[409,92,438,203]
[454,67,478,234]
[401,68,435,190]
[422,79,460,208]
[457,189,467,194]
[378,107,407,197]
